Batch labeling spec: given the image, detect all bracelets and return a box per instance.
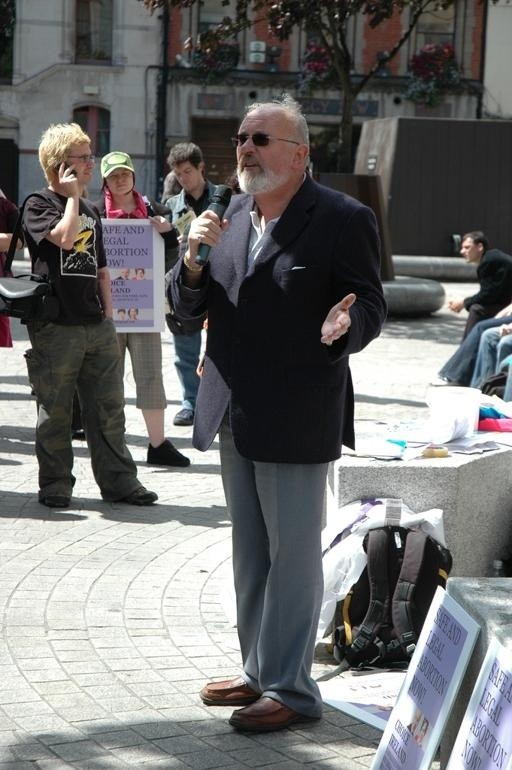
[184,255,203,273]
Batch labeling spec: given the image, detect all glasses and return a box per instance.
[231,131,300,147]
[59,154,94,164]
[107,171,131,180]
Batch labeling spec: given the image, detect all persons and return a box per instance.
[434,302,512,386]
[161,97,392,736]
[131,268,147,280]
[495,353,512,403]
[1,187,32,348]
[88,149,195,469]
[163,141,232,426]
[19,121,159,508]
[446,231,511,345]
[128,308,139,321]
[113,268,130,280]
[116,308,126,322]
[161,172,182,202]
[468,322,511,389]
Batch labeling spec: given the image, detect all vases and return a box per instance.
[221,44,241,68]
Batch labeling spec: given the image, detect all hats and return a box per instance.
[101,151,135,178]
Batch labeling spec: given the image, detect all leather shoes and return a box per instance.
[228,695,324,733]
[201,679,262,707]
[71,427,86,440]
[173,408,194,425]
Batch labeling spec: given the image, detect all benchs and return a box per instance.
[336,444,512,578]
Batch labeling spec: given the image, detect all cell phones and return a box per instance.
[54,162,78,177]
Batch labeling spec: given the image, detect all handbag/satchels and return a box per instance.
[0,273,61,323]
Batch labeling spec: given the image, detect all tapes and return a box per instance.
[423,447,449,458]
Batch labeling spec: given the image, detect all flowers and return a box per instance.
[297,47,336,96]
[191,31,229,83]
[404,43,462,104]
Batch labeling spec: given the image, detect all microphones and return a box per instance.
[194,185,233,266]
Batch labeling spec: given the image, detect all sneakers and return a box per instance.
[427,376,465,388]
[101,486,158,505]
[147,439,190,466]
[40,490,70,509]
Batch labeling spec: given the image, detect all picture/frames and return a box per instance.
[445,636,512,770]
[102,216,166,334]
[369,585,483,770]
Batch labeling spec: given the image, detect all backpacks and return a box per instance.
[329,524,453,670]
